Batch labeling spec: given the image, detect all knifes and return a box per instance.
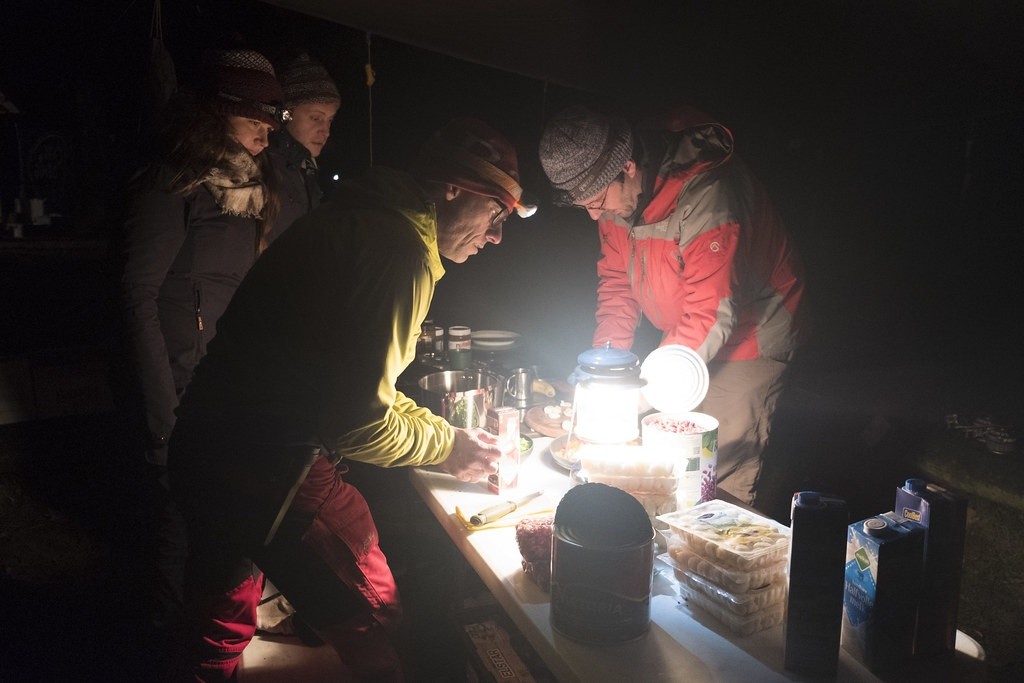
[468,489,543,524]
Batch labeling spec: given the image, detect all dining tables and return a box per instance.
[388,366,1024,683]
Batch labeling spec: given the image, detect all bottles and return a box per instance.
[894,477,965,657]
[486,405,520,493]
[419,326,444,350]
[448,324,472,349]
[841,511,925,678]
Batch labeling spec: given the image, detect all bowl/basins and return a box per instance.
[657,498,792,569]
[579,443,678,549]
[661,571,785,635]
[657,552,788,613]
[519,434,534,456]
[657,531,790,589]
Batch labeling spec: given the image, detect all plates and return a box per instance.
[550,436,642,470]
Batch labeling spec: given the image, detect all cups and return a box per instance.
[508,367,531,399]
[451,348,473,367]
[574,342,644,444]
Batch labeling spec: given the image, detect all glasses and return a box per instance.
[574,182,612,210]
[490,197,509,227]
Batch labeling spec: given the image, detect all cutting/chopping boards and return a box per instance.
[526,403,576,436]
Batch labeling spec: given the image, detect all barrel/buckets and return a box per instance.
[549,482,656,648]
[418,368,505,472]
[644,410,718,508]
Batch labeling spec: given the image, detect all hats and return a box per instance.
[537,98,633,207]
[410,115,523,214]
[194,31,286,132]
[276,47,342,109]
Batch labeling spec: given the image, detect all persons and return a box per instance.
[256,43,342,255]
[168,115,524,683]
[117,30,286,652]
[539,101,812,507]
